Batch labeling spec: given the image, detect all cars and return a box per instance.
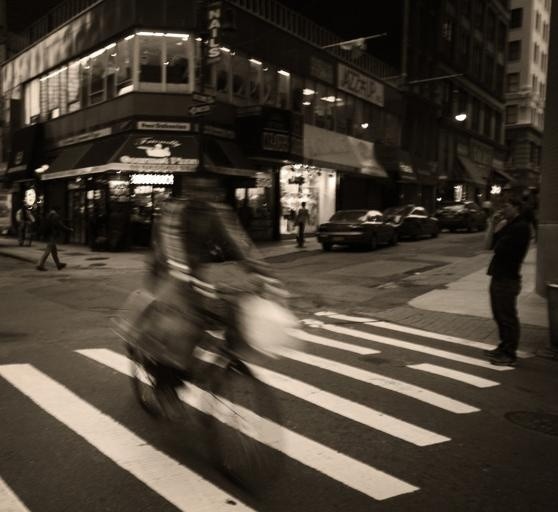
[309,199,489,253]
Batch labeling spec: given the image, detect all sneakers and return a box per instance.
[486,348,516,366]
[56,262,67,270]
[36,265,47,272]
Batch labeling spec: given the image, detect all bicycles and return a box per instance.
[123,268,298,495]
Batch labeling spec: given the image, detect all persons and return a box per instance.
[480,196,531,367]
[15,204,37,243]
[131,164,277,434]
[32,201,75,271]
[293,201,311,248]
